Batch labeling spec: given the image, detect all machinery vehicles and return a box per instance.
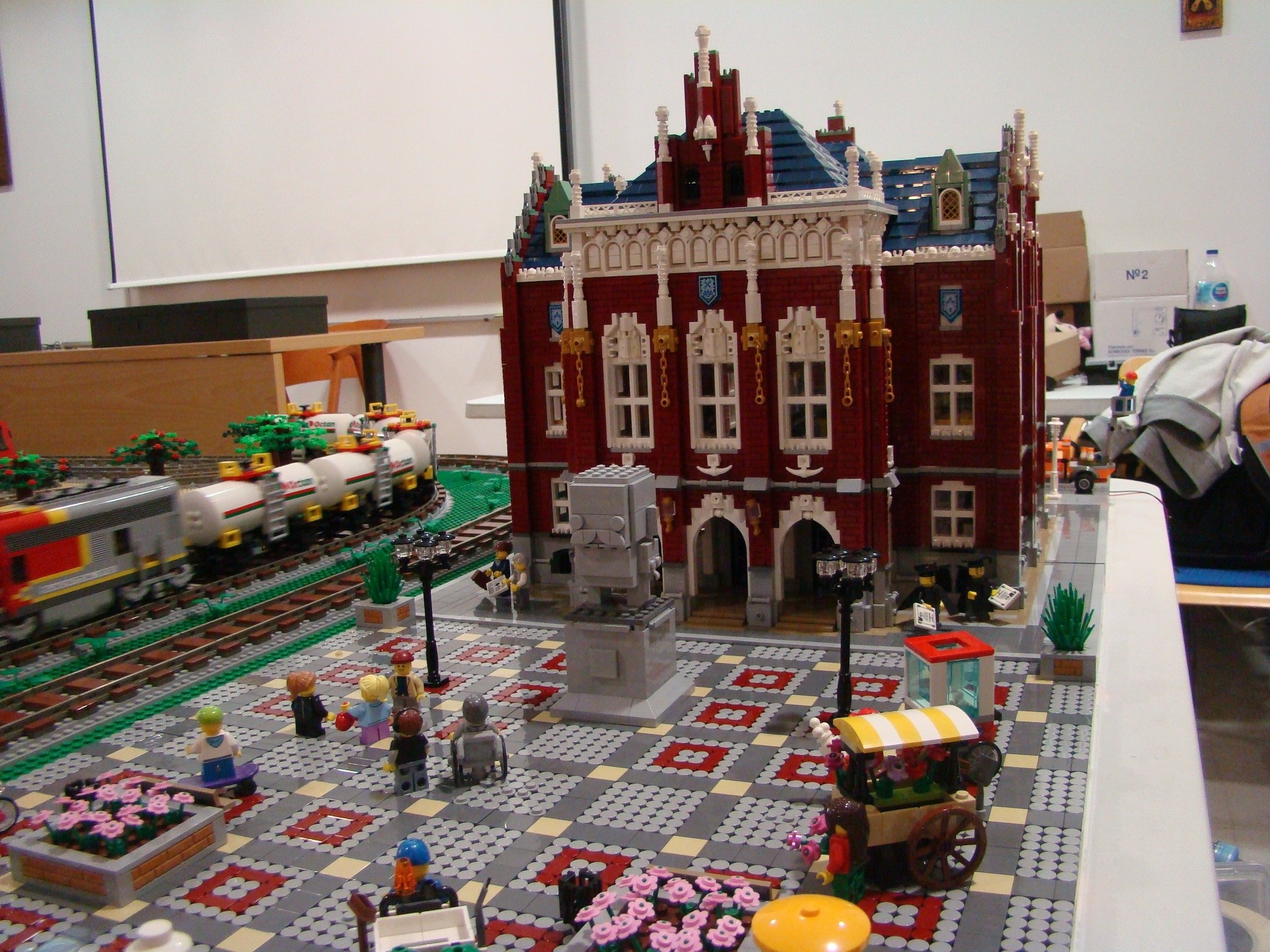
[355,876,493,952]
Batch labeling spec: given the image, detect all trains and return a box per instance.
[1,400,437,656]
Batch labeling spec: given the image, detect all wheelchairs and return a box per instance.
[449,729,511,788]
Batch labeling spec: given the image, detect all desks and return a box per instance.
[1043,384,1134,458]
[0,325,427,461]
[465,394,506,419]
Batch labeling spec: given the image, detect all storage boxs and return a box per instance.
[1035,211,1188,360]
[0,317,42,354]
[87,295,330,347]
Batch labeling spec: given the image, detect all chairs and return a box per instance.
[1117,357,1270,608]
[282,319,390,415]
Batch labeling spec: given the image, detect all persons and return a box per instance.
[447,693,500,743]
[897,556,998,636]
[185,706,239,782]
[1116,371,1137,411]
[816,796,870,904]
[485,539,531,612]
[342,650,430,796]
[380,838,451,909]
[286,671,335,737]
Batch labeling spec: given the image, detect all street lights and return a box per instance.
[809,543,882,729]
[1046,416,1064,499]
[389,528,457,689]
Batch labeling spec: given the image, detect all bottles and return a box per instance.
[1193,250,1232,310]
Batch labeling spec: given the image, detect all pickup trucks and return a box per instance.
[1045,441,1118,494]
